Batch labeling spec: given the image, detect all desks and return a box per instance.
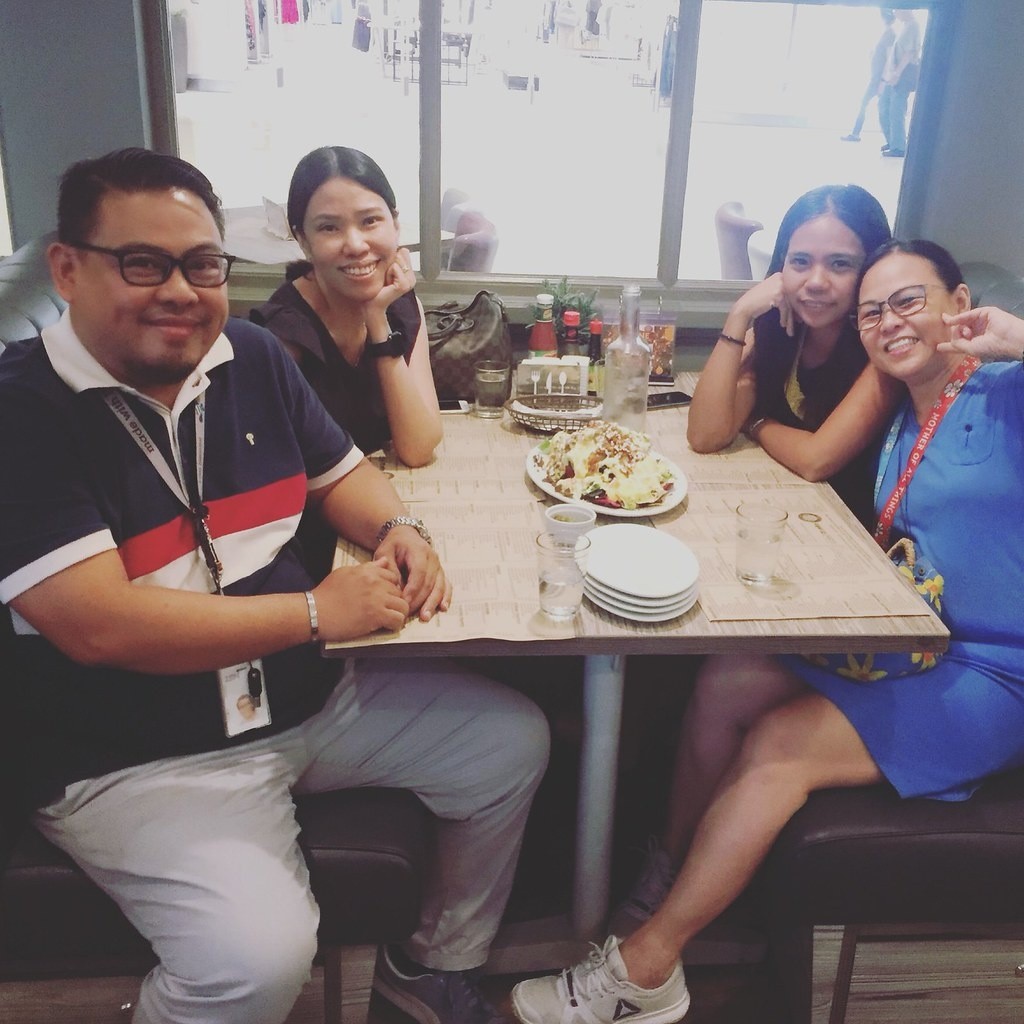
[217,203,454,272]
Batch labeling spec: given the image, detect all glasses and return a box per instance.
[845,283,945,331]
[68,240,236,287]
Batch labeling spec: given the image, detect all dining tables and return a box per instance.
[318,336,950,982]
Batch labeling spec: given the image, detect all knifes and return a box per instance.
[546,372,551,402]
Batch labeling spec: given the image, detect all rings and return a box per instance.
[402,264,409,274]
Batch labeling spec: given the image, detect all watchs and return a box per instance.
[373,331,406,359]
[374,514,432,546]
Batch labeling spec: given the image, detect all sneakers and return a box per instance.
[373,938,506,1024]
[624,835,682,922]
[509,934,691,1024]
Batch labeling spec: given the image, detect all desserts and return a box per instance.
[532,418,675,509]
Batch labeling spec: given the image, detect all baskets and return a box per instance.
[505,393,605,432]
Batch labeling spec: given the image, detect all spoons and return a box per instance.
[559,372,567,403]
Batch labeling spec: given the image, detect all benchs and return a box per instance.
[0,229,434,1024]
[760,263,1024,1024]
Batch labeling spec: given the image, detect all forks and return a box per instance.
[532,371,540,403]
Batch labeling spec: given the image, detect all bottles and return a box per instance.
[528,294,558,360]
[605,288,651,433]
[563,312,580,356]
[587,321,602,396]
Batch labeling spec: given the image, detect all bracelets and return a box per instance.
[749,417,766,438]
[720,333,746,347]
[305,590,318,642]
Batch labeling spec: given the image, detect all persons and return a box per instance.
[689,182,892,534]
[511,240,1024,1024]
[0,148,551,1024]
[840,8,921,158]
[236,693,266,727]
[248,144,443,584]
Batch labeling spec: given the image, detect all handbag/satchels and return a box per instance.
[423,290,511,397]
[805,538,943,681]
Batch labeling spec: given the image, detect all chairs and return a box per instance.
[441,187,475,234]
[446,211,499,272]
[746,228,776,281]
[715,201,763,281]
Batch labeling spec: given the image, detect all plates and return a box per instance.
[583,523,700,622]
[526,440,688,517]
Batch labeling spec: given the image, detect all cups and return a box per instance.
[736,504,789,586]
[473,360,510,418]
[546,504,597,536]
[535,530,591,619]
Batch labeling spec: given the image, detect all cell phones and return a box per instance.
[647,392,693,412]
